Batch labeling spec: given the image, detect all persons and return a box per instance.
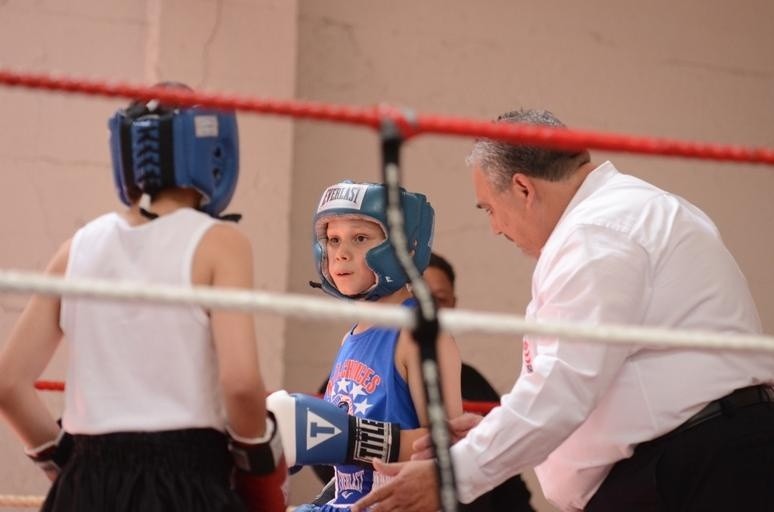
[2,76,290,512]
[347,109,773,512]
[252,178,468,512]
[302,243,535,511]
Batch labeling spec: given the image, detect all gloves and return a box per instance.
[222,407,288,512]
[265,387,401,472]
